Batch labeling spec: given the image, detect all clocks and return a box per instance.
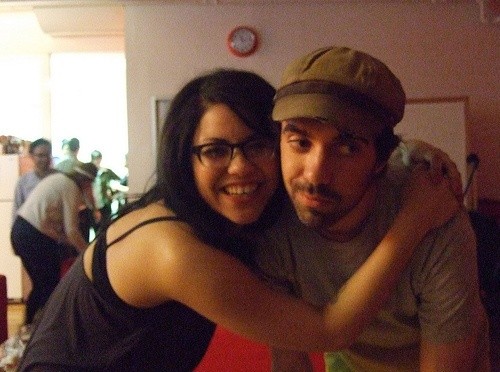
[226,26,257,57]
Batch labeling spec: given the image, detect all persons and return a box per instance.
[11,137,60,217]
[10,161,97,342]
[15,66,462,372]
[245,46,490,372]
[59,134,130,257]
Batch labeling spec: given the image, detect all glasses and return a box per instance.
[30,151,52,159]
[190,134,276,169]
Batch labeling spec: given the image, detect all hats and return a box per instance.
[272,45,406,131]
